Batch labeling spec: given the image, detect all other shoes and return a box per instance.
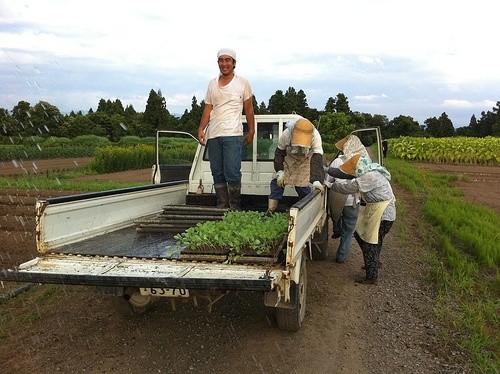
[358,278,378,285]
[362,262,384,269]
[331,231,343,239]
[336,258,344,263]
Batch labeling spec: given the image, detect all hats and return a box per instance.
[334,135,351,151]
[217,48,236,64]
[291,118,313,149]
[339,154,361,174]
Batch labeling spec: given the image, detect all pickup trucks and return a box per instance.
[1,112,385,332]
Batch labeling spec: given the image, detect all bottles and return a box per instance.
[197,179,205,194]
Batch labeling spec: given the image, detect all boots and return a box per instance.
[214,183,229,209]
[228,185,241,211]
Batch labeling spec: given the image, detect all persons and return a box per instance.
[198,48,255,212]
[382,138,388,159]
[267,118,326,213]
[327,134,372,263]
[322,154,397,286]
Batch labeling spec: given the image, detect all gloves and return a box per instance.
[276,170,286,187]
[322,165,329,172]
[312,181,325,194]
[323,181,333,188]
